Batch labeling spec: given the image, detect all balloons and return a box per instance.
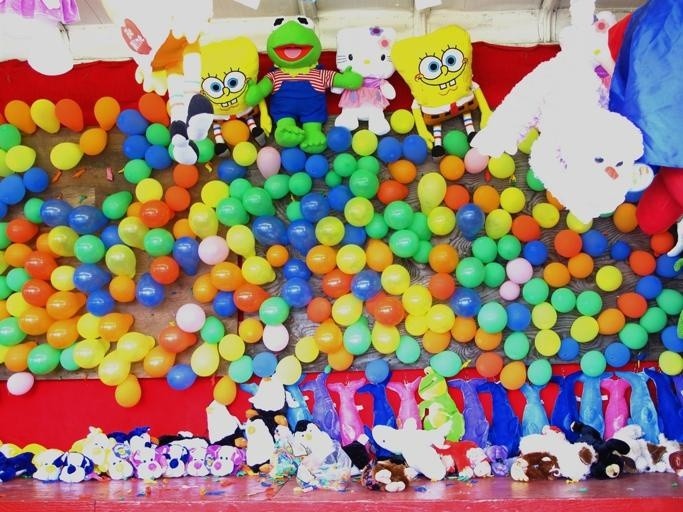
[1,95,683,403]
[0,94,683,403]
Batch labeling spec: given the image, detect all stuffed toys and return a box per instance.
[0,366,683,492]
[2,366,683,493]
[102,0,213,165]
[335,27,396,136]
[608,1,683,258]
[202,37,273,166]
[390,24,496,162]
[470,0,655,223]
[245,16,363,151]
[1,0,81,77]
